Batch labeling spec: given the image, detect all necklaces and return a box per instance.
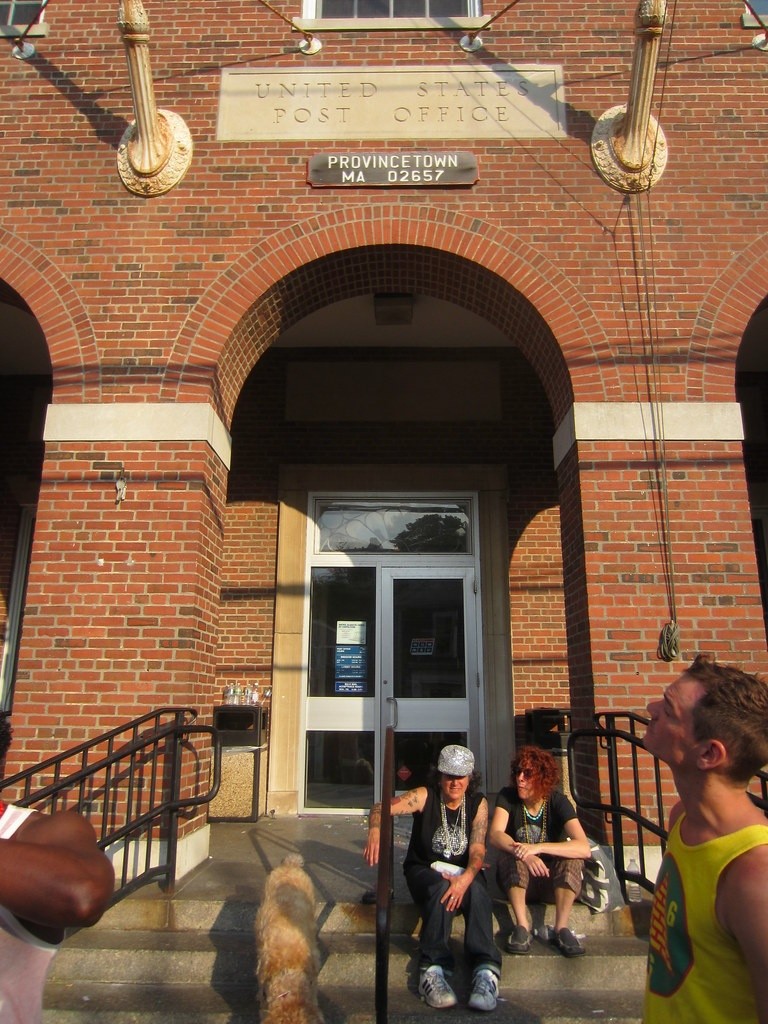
[439,786,469,856]
[520,796,549,860]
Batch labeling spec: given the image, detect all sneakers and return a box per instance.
[418,971,457,1008]
[467,968,500,1010]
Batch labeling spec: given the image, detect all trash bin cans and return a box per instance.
[208,705,267,823]
[524,708,577,817]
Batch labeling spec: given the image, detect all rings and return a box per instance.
[454,899,458,902]
[450,896,454,900]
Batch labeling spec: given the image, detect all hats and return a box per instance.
[437,745,475,777]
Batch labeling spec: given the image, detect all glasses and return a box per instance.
[512,766,535,780]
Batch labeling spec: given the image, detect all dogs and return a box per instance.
[253,853,327,1024]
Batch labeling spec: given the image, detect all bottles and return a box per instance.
[627,857,641,902]
[223,681,273,707]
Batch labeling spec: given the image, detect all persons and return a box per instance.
[488,745,594,959]
[0,710,119,1024]
[364,743,501,1012]
[640,653,768,1022]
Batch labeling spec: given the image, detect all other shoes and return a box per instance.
[553,927,587,958]
[507,924,536,954]
